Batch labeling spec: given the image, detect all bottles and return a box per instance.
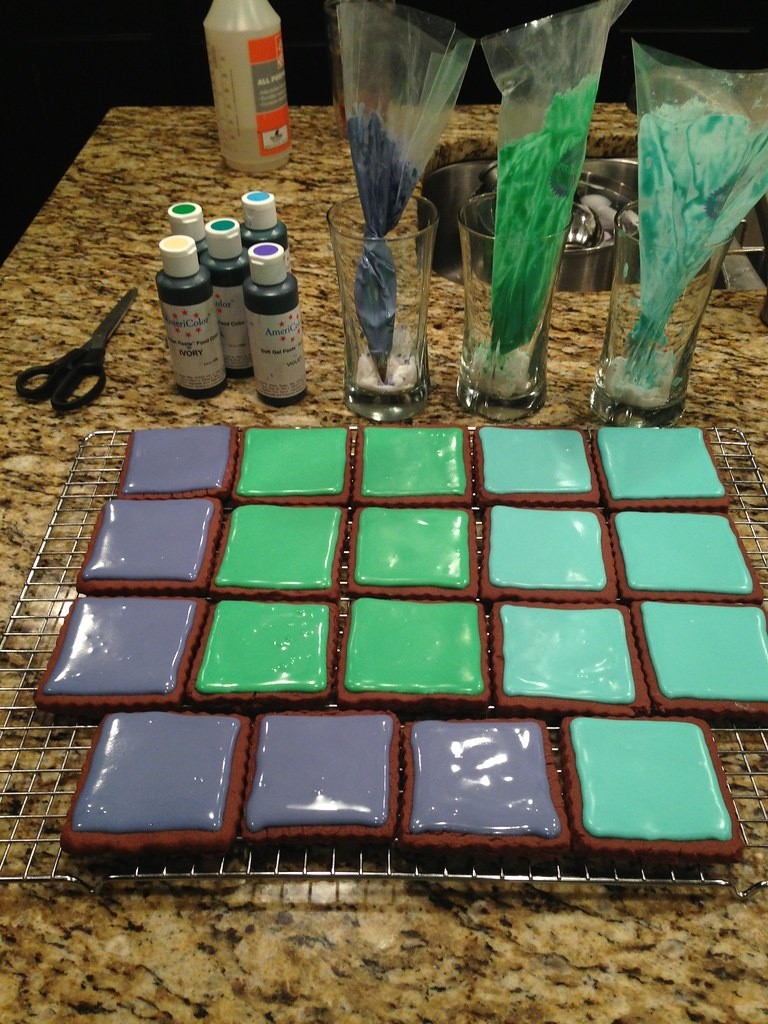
[204,0,291,174]
[155,191,306,407]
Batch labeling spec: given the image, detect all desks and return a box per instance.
[0,102,767,1024]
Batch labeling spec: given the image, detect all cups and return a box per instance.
[456,190,573,420]
[590,203,734,428]
[326,192,441,423]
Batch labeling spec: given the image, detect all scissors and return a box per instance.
[16,287,139,412]
